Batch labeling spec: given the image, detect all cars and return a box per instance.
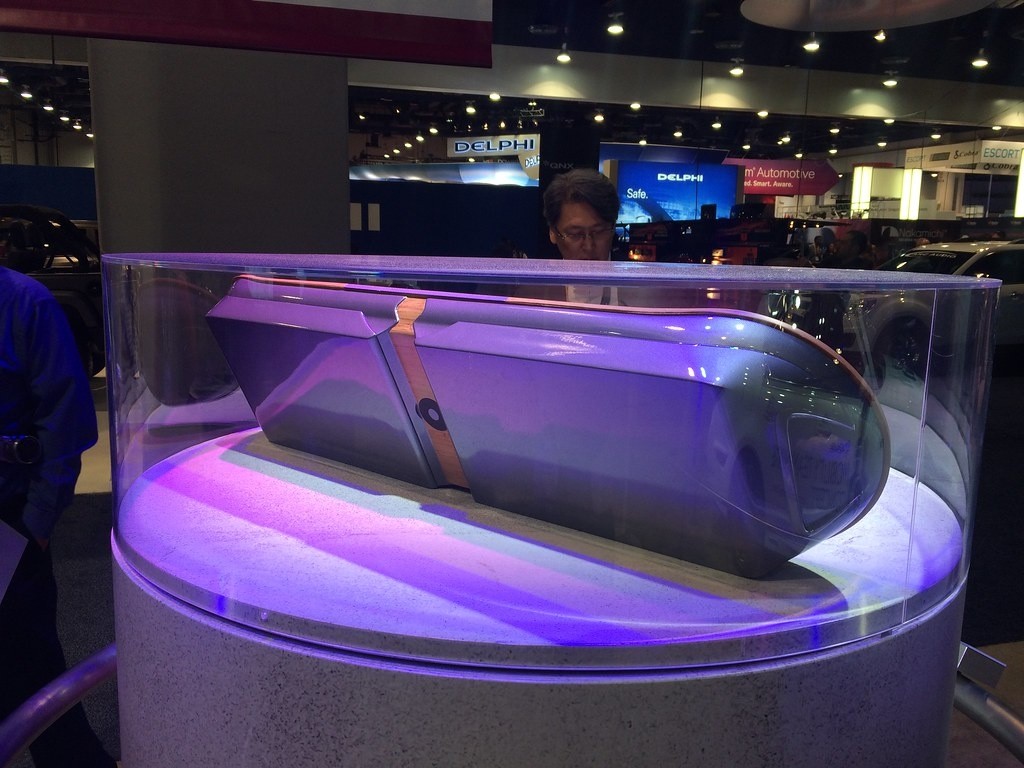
[0,204,103,381]
[760,241,1024,381]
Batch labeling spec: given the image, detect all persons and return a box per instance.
[1,268,115,768]
[515,166,690,309]
[808,236,834,262]
[806,230,874,270]
[765,246,812,267]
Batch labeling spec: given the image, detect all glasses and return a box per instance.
[553,222,618,245]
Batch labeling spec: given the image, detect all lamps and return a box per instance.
[0,11,1024,163]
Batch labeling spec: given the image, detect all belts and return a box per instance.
[0,435,44,466]
